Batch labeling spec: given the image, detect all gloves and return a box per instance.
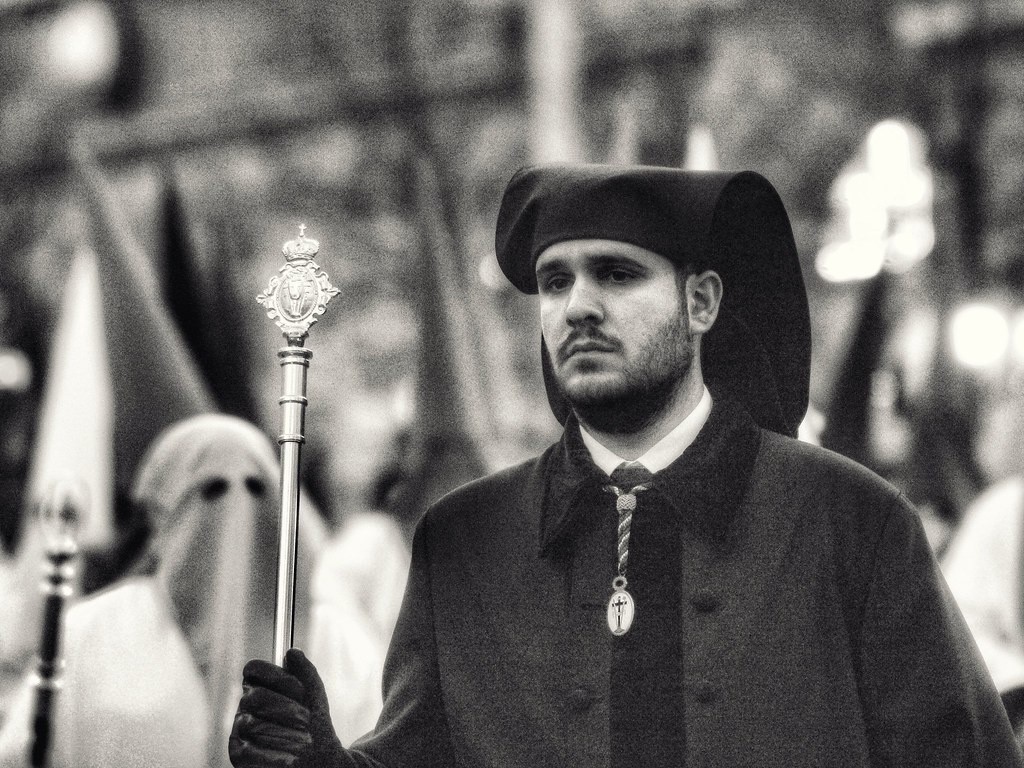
[229,648,341,768]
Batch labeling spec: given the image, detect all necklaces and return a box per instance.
[602,481,655,635]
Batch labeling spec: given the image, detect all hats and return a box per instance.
[493,162,811,435]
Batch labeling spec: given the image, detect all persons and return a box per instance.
[1,416,329,768]
[229,160,1024,768]
